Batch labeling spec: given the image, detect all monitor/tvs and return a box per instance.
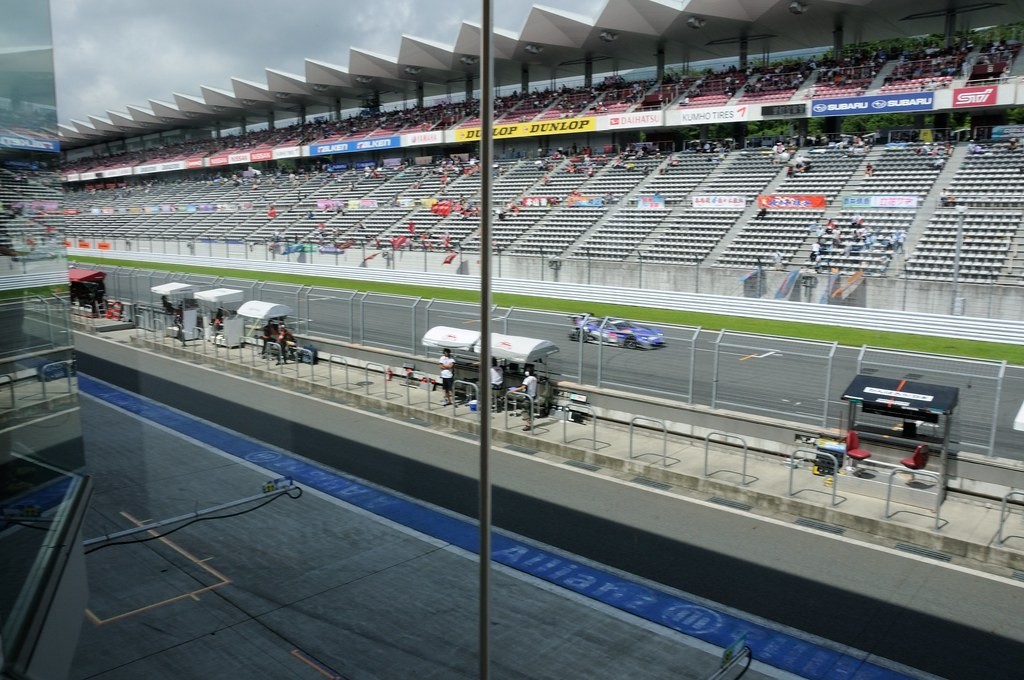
[570,393,587,403]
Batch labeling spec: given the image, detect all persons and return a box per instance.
[509,366,538,432]
[213,306,225,330]
[438,345,458,407]
[489,355,504,391]
[177,299,185,326]
[0,25,1021,301]
[275,321,288,366]
[161,295,177,313]
[259,317,277,359]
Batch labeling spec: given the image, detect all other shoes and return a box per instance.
[522,426,531,431]
[275,362,281,365]
[443,400,449,406]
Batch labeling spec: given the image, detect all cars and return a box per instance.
[569,314,665,349]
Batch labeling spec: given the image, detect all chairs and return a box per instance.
[900,443,930,485]
[846,431,872,473]
